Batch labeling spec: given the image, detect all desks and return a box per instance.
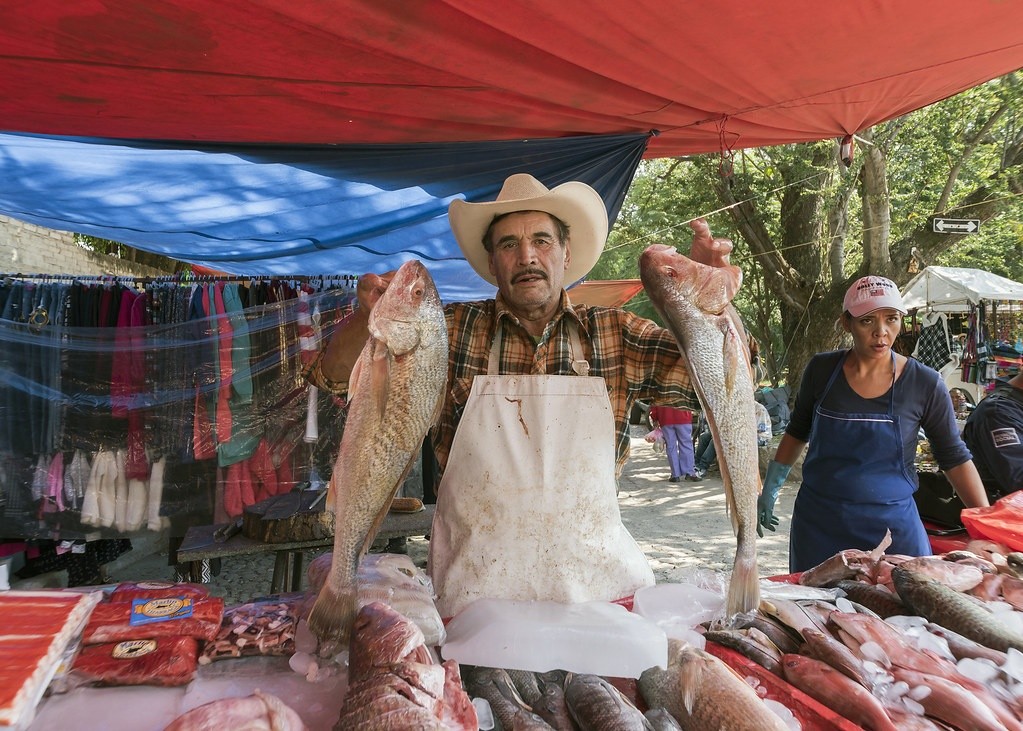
[177,504,436,596]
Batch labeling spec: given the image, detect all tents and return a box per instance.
[899,265,1023,344]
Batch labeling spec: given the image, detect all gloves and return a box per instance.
[756,459,793,538]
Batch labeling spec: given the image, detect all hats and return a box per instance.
[843,275,909,319]
[449,173,609,290]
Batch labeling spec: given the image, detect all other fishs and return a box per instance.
[306,257,450,644]
[638,243,762,617]
[337,547,1023,731]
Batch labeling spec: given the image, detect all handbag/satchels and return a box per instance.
[960,342,1000,384]
[960,489,1023,552]
[643,425,665,454]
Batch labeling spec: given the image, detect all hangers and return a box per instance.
[0,272,362,293]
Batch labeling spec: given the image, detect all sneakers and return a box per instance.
[695,471,707,477]
[685,473,702,482]
[693,465,701,472]
[669,475,680,482]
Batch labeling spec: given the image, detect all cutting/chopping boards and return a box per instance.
[242,496,335,543]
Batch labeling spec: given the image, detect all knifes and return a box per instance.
[262,479,312,521]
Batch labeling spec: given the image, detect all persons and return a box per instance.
[756,275,992,575]
[305,173,765,619]
[381,536,409,554]
[949,387,976,420]
[961,368,1023,504]
[695,399,773,477]
[650,406,702,482]
